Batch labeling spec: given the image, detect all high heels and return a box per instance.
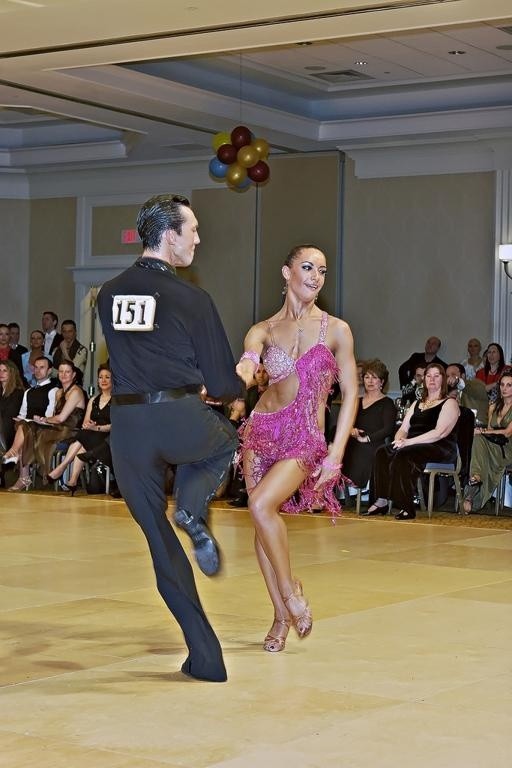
[45,474,62,482]
[7,476,32,493]
[2,449,19,464]
[58,484,76,496]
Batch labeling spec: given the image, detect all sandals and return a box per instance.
[264,614,293,652]
[283,578,312,636]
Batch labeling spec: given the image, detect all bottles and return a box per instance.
[395,397,410,421]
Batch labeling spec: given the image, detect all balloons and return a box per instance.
[208,126,270,188]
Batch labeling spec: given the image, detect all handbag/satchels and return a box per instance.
[482,426,509,446]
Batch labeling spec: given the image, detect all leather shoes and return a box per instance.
[226,491,247,507]
[174,509,221,576]
[396,510,415,520]
[359,503,389,516]
[76,453,97,466]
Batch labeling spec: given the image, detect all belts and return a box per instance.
[111,384,205,405]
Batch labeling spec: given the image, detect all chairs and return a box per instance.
[327,388,511,519]
[24,442,111,493]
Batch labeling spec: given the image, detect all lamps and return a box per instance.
[497,242,512,279]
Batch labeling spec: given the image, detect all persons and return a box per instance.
[0,312,123,500]
[229,245,359,653]
[96,193,248,683]
[230,358,271,508]
[327,337,512,520]
